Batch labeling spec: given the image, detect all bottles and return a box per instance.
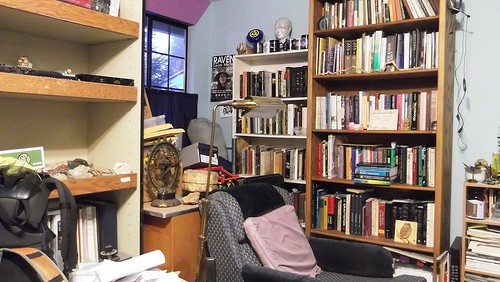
[246,29,264,54]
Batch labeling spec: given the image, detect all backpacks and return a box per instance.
[0,164,79,282]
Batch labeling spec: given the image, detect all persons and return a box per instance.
[213,69,233,100]
[275,18,292,46]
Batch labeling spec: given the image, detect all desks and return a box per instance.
[142,202,204,282]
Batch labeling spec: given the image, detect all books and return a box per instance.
[314,0,500,282]
[237,65,308,225]
[45,202,99,273]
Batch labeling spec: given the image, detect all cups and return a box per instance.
[263,34,308,53]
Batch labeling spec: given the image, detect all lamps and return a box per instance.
[194,97,284,282]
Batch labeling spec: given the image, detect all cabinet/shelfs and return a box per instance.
[0,0,143,275]
[233,49,309,234]
[460,182,500,282]
[306,0,456,282]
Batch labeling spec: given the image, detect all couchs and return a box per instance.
[198,185,427,282]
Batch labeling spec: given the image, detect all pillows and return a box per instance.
[245,205,322,278]
